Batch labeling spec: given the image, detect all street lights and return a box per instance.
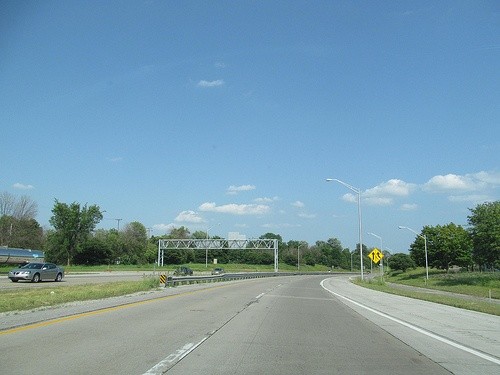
[326,178,365,279]
[398,225,431,281]
[367,232,384,281]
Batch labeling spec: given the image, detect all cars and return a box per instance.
[9,262,65,282]
[172,267,193,277]
[212,268,225,274]
